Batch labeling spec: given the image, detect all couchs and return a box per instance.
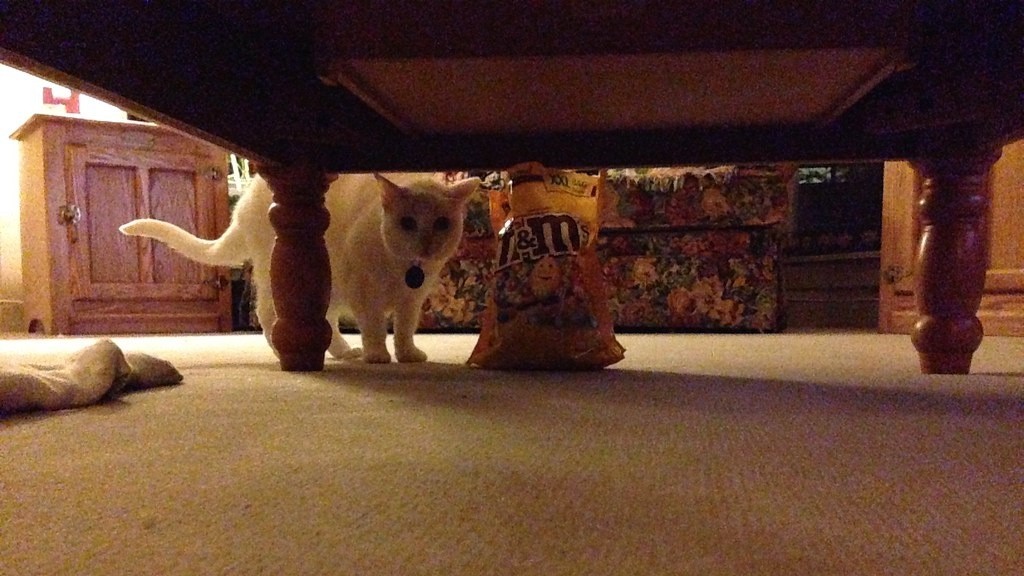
[339,164,794,333]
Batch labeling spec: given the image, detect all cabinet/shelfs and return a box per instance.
[10,113,234,334]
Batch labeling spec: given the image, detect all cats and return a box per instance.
[120,170,481,364]
